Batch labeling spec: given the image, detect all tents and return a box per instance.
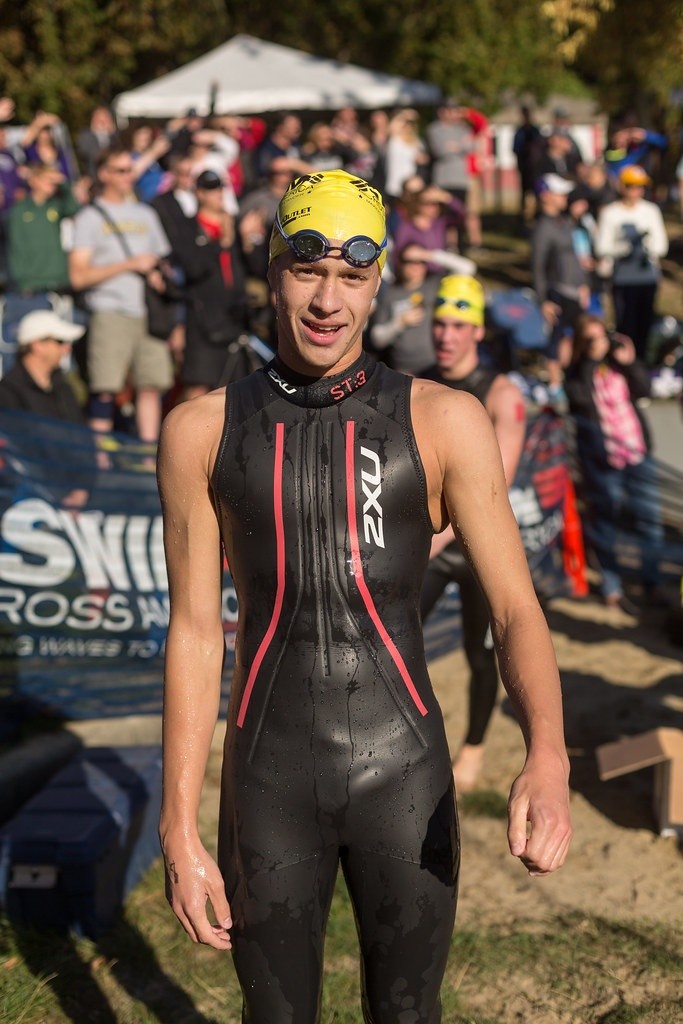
[113,34,443,116]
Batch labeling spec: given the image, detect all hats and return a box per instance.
[432,274,487,326]
[268,168,387,275]
[16,308,87,345]
[554,105,574,118]
[197,170,223,189]
[27,162,66,185]
[532,173,577,195]
[618,164,650,186]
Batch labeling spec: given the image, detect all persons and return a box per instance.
[0,99,683,798]
[158,167,575,1024]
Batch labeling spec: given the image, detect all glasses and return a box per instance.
[116,167,132,174]
[286,229,384,269]
[434,296,473,310]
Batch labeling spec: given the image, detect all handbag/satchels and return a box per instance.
[144,288,177,339]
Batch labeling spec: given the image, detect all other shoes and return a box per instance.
[605,592,629,611]
[638,583,677,608]
